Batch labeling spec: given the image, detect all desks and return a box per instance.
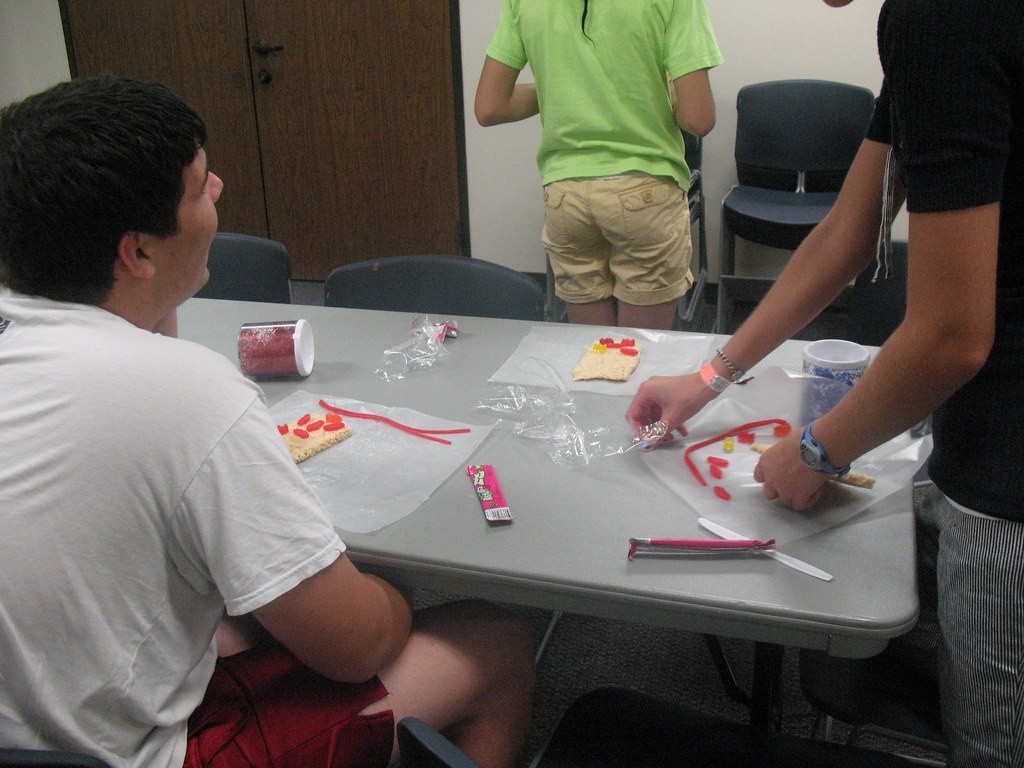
[177,299,915,724]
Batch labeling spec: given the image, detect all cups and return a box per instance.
[801,340,870,425]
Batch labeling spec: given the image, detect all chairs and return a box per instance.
[192,230,294,303]
[322,79,899,335]
[394,634,949,768]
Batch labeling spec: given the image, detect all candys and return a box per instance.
[706,424,792,500]
[592,337,639,354]
[277,413,346,439]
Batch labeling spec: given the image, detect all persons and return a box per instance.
[624,0,1022,767]
[1,71,533,768]
[475,0,725,330]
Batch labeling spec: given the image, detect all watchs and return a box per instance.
[799,422,851,480]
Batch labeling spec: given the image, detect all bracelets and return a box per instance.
[700,360,732,393]
[715,347,745,382]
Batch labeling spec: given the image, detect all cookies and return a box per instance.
[751,444,877,490]
[276,412,352,465]
[572,337,641,380]
